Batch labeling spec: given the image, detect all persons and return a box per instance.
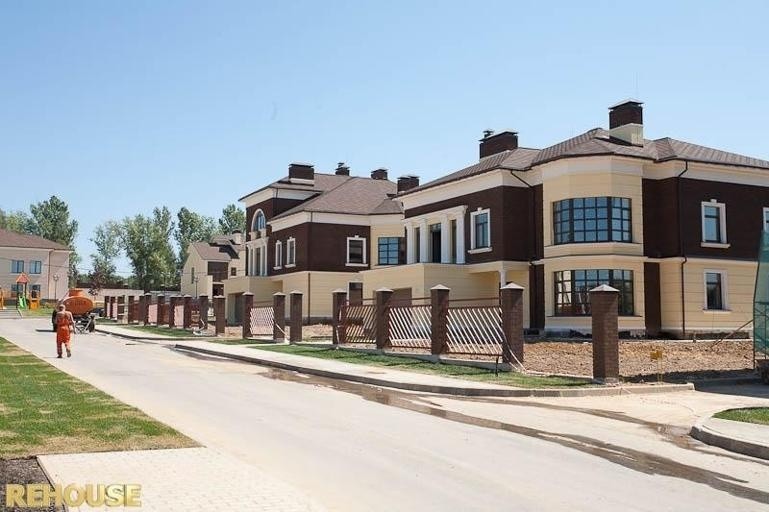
[54,303,76,358]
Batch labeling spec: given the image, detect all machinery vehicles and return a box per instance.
[51,286,96,333]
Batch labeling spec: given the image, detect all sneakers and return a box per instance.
[56,351,71,358]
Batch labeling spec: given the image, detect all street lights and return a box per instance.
[161,271,171,296]
[194,275,200,299]
